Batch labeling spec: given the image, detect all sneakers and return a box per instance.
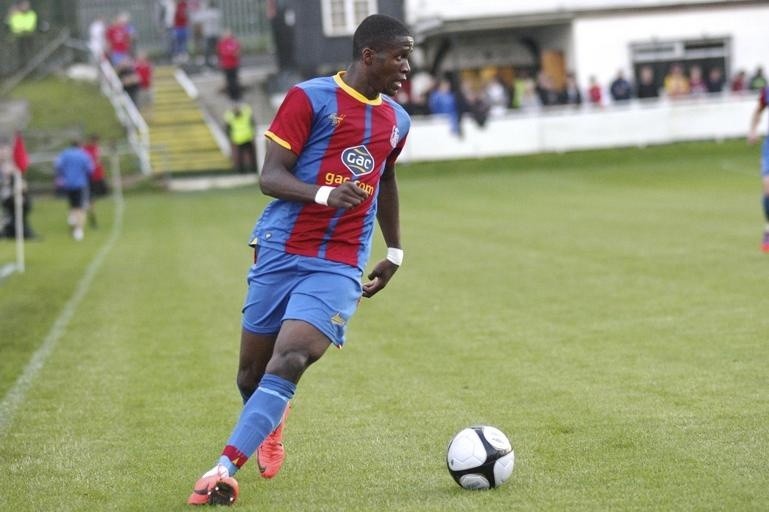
[187,472,239,506]
[256,404,291,479]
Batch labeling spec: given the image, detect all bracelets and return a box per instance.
[316,180,338,209]
[385,245,407,270]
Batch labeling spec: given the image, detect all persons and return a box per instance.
[84,135,113,229]
[53,132,97,242]
[5,2,40,73]
[222,96,258,174]
[85,1,224,106]
[215,23,241,92]
[746,83,769,257]
[558,63,690,106]
[180,13,415,504]
[690,64,765,94]
[396,70,559,116]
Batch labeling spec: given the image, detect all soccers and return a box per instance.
[445,425,514,488]
